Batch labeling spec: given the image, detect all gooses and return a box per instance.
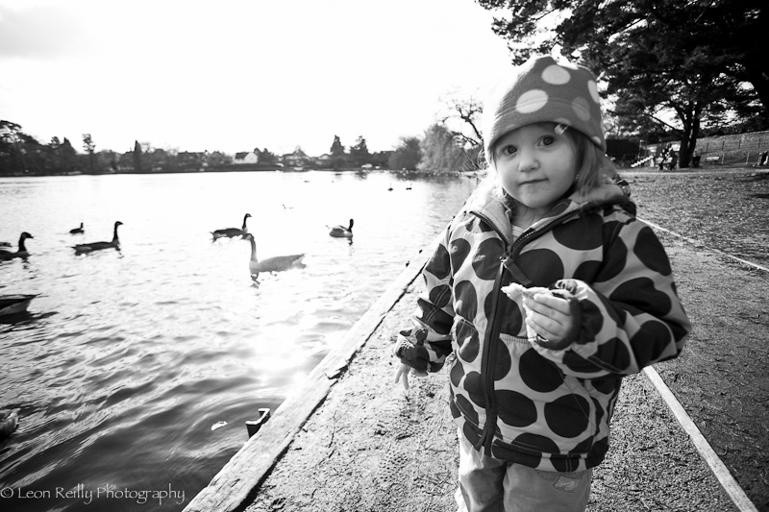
[71,220,124,252]
[329,218,355,238]
[209,213,254,239]
[70,222,85,233]
[0,292,41,315]
[242,232,306,272]
[0,230,34,261]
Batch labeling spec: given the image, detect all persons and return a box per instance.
[392,50,691,512]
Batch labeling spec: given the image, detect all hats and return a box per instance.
[487,56,606,151]
